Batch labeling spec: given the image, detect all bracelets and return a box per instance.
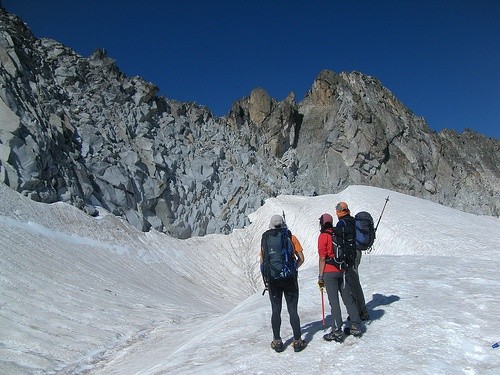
[319,274,324,279]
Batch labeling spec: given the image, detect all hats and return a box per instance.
[318,213,333,226]
[335,202,349,212]
[270,215,283,227]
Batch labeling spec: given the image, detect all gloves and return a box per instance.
[317,276,325,291]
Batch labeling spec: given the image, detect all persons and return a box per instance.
[318,212,361,342]
[259,214,307,353]
[334,201,370,322]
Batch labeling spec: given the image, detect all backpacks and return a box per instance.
[260,228,298,281]
[321,228,356,270]
[339,211,375,251]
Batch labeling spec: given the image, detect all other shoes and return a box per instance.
[344,323,363,338]
[347,308,370,321]
[323,328,344,343]
[293,339,307,352]
[270,337,284,352]
